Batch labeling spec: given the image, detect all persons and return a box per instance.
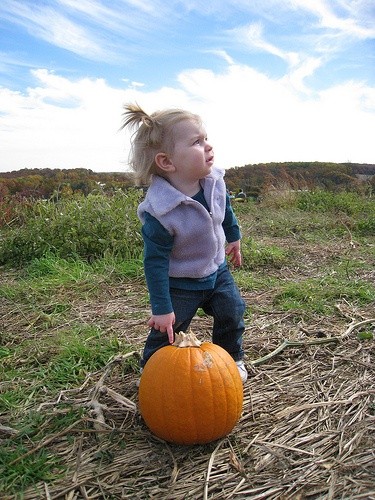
[226,189,246,202]
[119,101,248,388]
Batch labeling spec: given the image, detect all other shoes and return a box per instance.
[235,360,248,384]
[136,367,144,387]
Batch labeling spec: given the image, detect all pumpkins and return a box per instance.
[139,327,243,443]
